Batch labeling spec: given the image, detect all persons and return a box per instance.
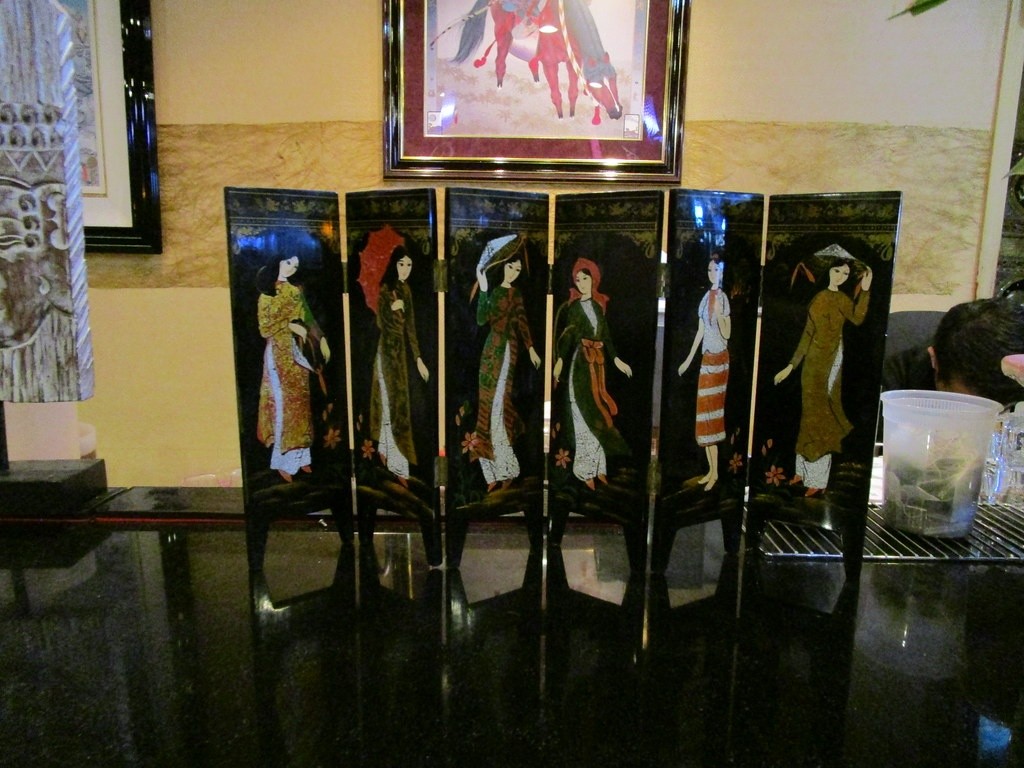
[875,297,1024,455]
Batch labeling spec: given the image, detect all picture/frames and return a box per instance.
[60,0,164,256]
[379,0,690,187]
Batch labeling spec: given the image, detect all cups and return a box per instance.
[984,415,1024,506]
[77,423,97,460]
[879,390,1004,539]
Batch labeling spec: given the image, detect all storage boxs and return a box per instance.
[0,454,106,512]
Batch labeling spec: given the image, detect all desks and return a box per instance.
[0,482,1024,768]
